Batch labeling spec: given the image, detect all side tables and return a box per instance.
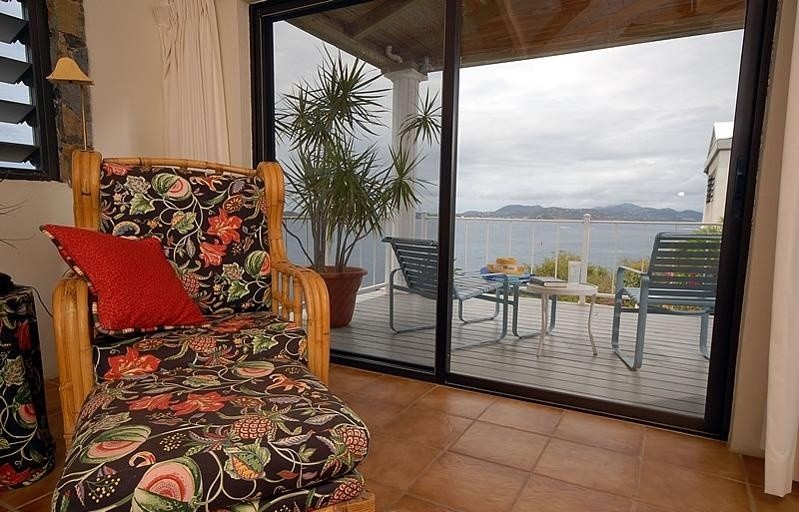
[527,278,598,357]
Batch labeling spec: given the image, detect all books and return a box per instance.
[530,276,567,288]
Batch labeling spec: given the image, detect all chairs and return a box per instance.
[612,231,723,371]
[53,149,377,512]
[381,236,557,351]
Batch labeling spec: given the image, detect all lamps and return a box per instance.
[46,56,95,150]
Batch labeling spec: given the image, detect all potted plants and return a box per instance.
[272,42,441,328]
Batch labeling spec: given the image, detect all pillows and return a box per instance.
[38,222,207,335]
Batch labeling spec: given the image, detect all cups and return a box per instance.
[568,261,581,286]
